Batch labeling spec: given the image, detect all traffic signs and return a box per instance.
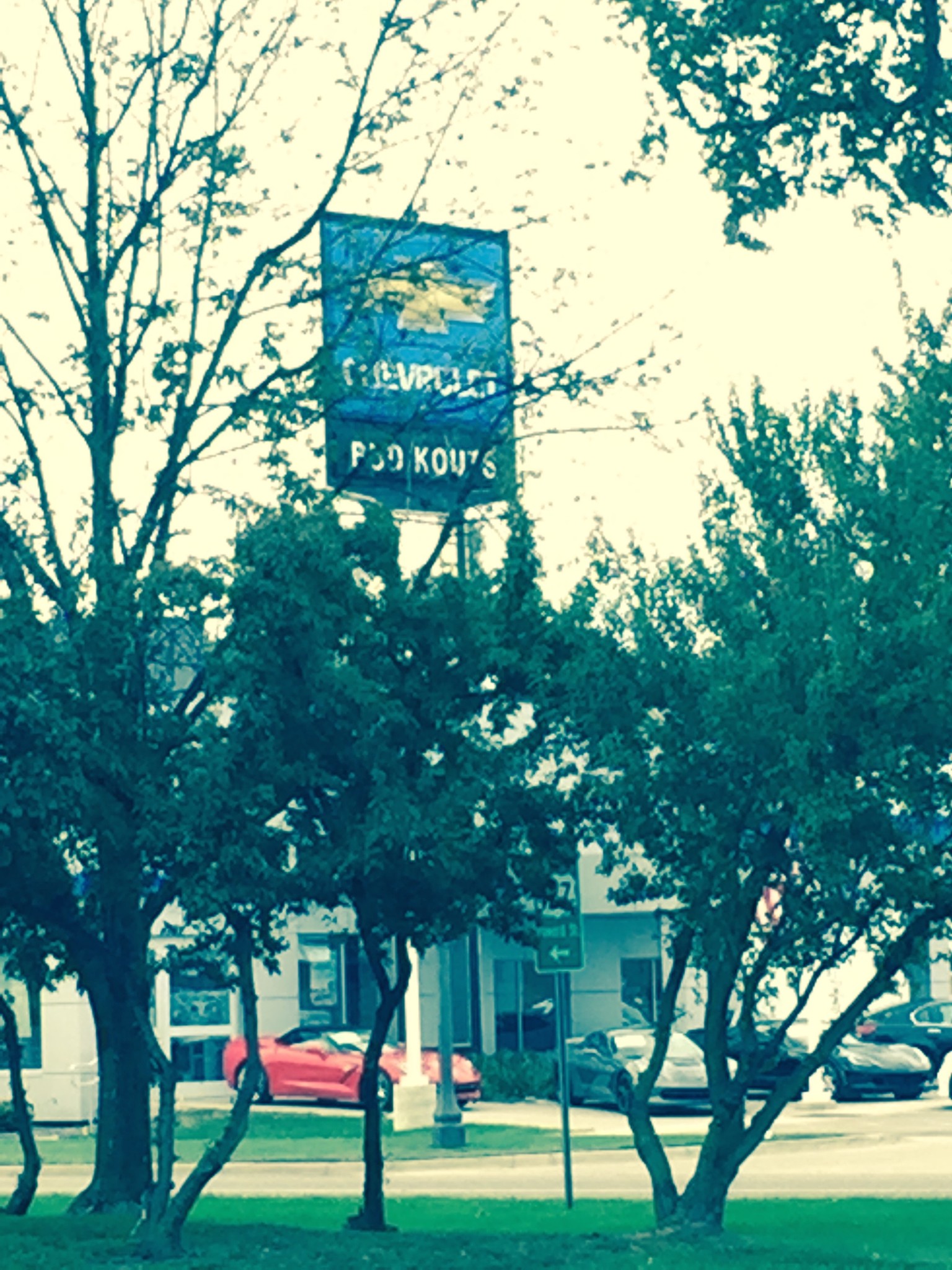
[534,853,587,972]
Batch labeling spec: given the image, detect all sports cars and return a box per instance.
[559,1031,744,1113]
[693,1017,930,1102]
[222,1025,480,1110]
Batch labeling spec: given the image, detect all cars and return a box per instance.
[860,999,952,1081]
